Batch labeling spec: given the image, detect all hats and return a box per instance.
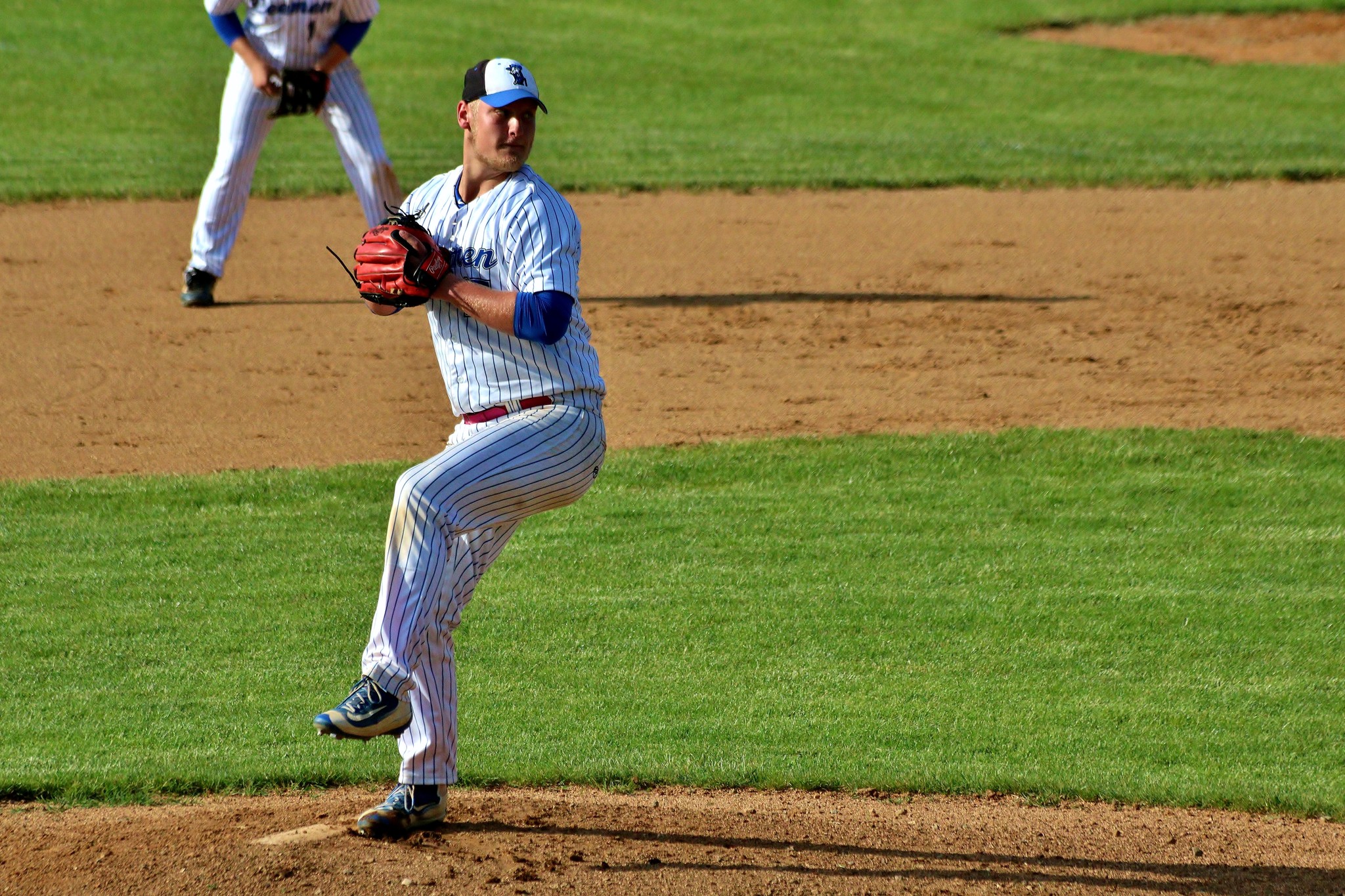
[462,57,547,115]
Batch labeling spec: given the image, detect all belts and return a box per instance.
[464,396,553,425]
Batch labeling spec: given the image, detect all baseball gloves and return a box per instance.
[352,213,452,308]
[265,64,329,121]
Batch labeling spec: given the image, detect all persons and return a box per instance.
[181,0,405,307]
[313,57,605,837]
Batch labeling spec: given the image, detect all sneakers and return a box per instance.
[358,781,448,838]
[312,678,412,742]
[180,269,216,306]
